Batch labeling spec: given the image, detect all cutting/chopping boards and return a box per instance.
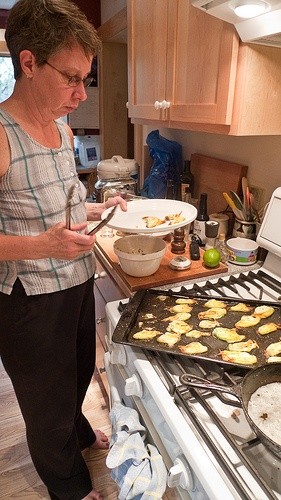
[86,222,229,291]
[190,153,249,223]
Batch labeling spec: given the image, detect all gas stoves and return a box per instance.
[103,185,280,500]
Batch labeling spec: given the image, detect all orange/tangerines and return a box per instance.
[203,248,221,268]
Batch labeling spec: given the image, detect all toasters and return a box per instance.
[78,136,100,168]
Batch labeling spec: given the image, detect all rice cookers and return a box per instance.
[97,155,139,180]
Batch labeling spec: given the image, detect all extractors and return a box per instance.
[191,0,281,48]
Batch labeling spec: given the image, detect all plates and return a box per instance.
[101,199,198,234]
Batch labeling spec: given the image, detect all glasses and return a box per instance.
[29,49,94,88]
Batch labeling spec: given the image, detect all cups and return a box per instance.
[209,213,230,238]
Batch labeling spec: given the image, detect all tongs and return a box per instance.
[65,185,118,236]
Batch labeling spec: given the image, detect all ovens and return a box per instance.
[103,352,191,499]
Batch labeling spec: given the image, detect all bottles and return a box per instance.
[175,159,194,204]
[94,172,137,203]
[193,193,209,246]
[215,233,230,264]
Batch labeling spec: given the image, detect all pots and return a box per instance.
[178,361,280,451]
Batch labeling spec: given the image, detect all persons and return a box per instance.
[0,0,129,500]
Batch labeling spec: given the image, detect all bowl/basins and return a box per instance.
[226,237,259,266]
[113,235,167,277]
[233,216,258,240]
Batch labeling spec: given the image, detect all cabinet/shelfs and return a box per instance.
[167,0,280,136]
[91,253,129,409]
[125,0,167,127]
